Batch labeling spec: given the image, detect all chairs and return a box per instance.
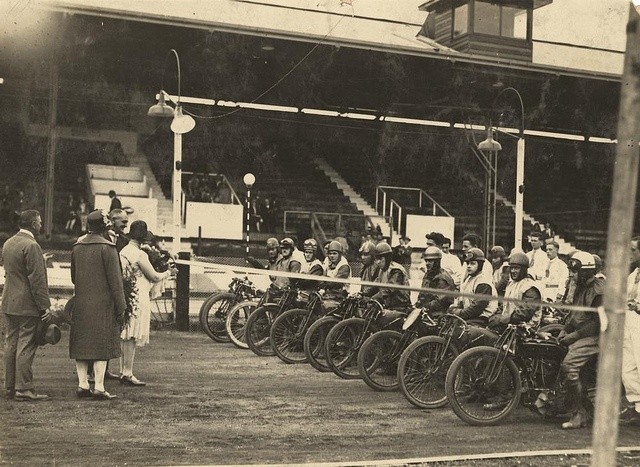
[0,121,128,231]
[390,148,610,256]
[142,129,391,256]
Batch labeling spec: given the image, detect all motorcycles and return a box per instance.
[357,294,468,393]
[268,276,361,365]
[303,282,403,373]
[396,306,532,409]
[445,299,637,425]
[226,276,307,351]
[246,275,327,358]
[325,289,429,379]
[200,277,275,344]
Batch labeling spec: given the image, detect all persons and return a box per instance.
[399,236,413,280]
[317,241,352,301]
[366,233,372,241]
[0,207,62,400]
[443,232,494,290]
[488,251,542,336]
[489,245,509,292]
[358,239,379,298]
[369,229,378,245]
[118,220,180,387]
[592,253,606,279]
[543,222,553,238]
[105,209,128,251]
[69,210,128,400]
[321,239,332,269]
[618,236,640,426]
[540,240,569,303]
[357,241,412,311]
[140,236,171,274]
[245,237,282,269]
[378,233,387,243]
[533,222,541,231]
[76,195,89,230]
[524,231,547,279]
[273,238,301,290]
[106,190,123,212]
[63,194,77,231]
[296,238,324,291]
[391,237,403,263]
[418,231,461,274]
[2,183,44,233]
[415,245,456,312]
[442,237,461,266]
[554,250,606,430]
[188,168,231,205]
[451,247,499,320]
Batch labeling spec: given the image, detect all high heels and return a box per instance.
[120,375,146,386]
[76,386,93,398]
[93,390,117,400]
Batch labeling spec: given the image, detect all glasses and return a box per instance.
[359,248,370,253]
[304,240,316,246]
[567,258,595,269]
[463,250,482,260]
[491,249,506,253]
[281,240,292,245]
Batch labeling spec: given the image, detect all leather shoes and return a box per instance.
[561,406,588,429]
[87,374,95,383]
[483,393,512,411]
[14,390,48,401]
[6,389,13,399]
[105,372,119,379]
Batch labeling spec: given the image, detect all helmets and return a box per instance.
[375,242,392,256]
[328,241,343,252]
[465,248,485,262]
[334,237,348,250]
[508,254,529,267]
[359,241,375,255]
[266,238,279,249]
[423,246,442,259]
[280,238,294,250]
[568,251,596,268]
[304,239,317,250]
[491,246,506,257]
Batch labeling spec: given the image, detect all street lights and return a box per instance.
[148,48,181,322]
[477,86,525,253]
[242,172,256,267]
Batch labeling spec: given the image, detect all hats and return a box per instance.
[125,220,155,242]
[36,315,62,346]
[403,237,411,242]
[86,210,114,232]
